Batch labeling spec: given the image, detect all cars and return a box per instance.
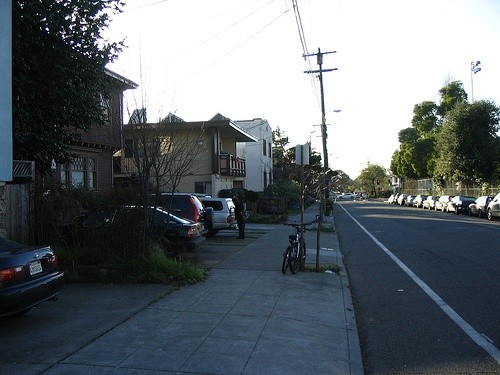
[446,195,477,215]
[467,196,495,219]
[485,192,500,221]
[337,193,358,199]
[199,194,240,240]
[434,195,456,212]
[387,193,408,206]
[412,195,431,208]
[404,195,417,207]
[55,206,208,259]
[421,195,440,210]
[0,236,65,327]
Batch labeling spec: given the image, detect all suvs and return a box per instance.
[138,192,215,239]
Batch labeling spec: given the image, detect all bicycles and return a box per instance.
[281,221,313,274]
